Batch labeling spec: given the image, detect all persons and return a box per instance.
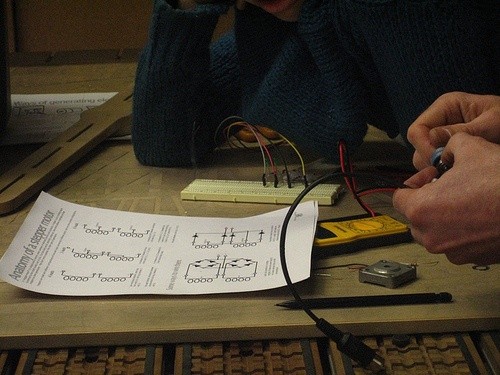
[130,1,500,168]
[391,90,500,267]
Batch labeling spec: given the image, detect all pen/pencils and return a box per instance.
[273,291,453,307]
